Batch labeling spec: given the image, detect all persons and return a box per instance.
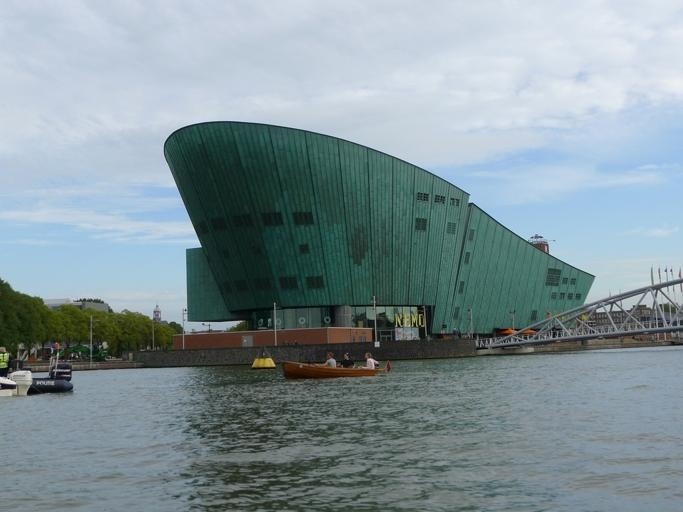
[0,346,14,377]
[336,351,356,368]
[358,352,378,370]
[318,351,337,369]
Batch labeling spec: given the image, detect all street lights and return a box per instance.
[182,307,188,350]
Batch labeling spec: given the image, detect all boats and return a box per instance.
[283,360,391,379]
[0,342,73,397]
